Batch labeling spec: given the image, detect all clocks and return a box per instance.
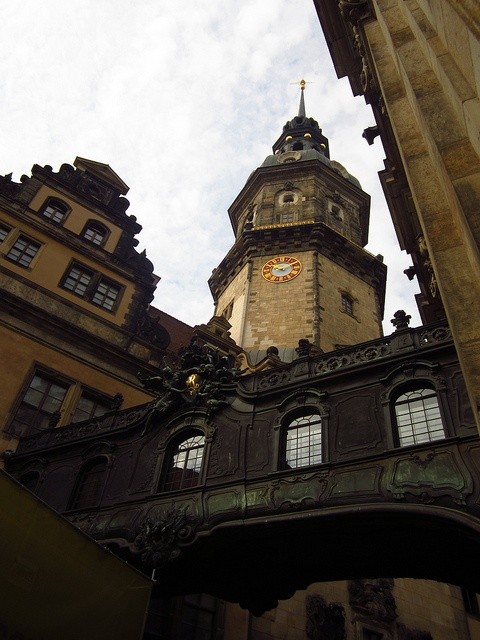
[261,256,302,283]
